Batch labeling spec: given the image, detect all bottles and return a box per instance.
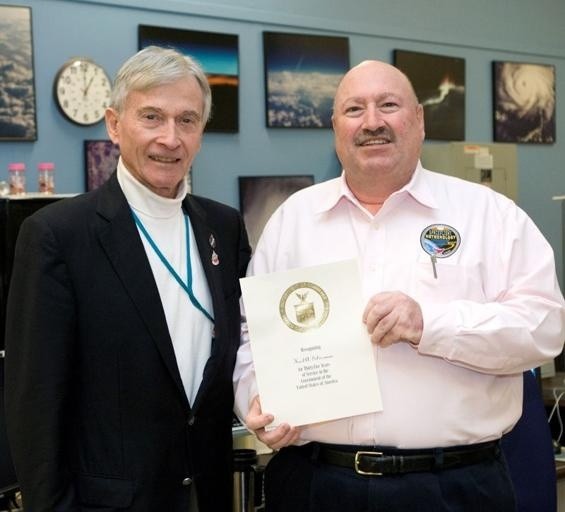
[7,162,25,195]
[38,164,55,193]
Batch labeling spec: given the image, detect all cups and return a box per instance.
[233,449,262,512]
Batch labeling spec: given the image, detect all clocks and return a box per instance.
[54,56,114,126]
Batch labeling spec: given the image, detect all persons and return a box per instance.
[232,60,565,512]
[4,45,251,512]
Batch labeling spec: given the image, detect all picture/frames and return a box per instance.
[237,175,315,254]
[0,2,39,142]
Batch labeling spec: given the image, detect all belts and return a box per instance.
[299,443,496,477]
